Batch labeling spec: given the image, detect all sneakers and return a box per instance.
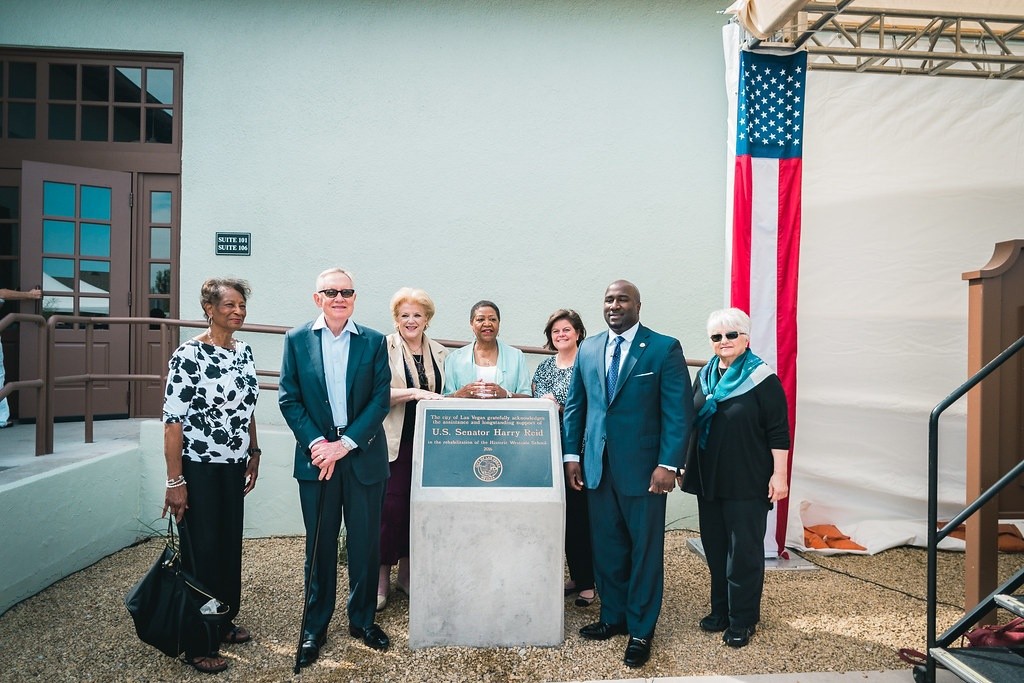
[700,613,729,631]
[724,624,756,646]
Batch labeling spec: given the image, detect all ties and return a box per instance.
[607,336,625,405]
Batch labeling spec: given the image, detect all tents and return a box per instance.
[43,272,110,314]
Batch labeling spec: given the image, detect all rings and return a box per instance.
[494,391,497,397]
[471,391,473,395]
[320,455,323,459]
[663,490,668,492]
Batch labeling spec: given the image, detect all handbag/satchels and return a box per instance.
[124,514,232,663]
[899,616,1024,664]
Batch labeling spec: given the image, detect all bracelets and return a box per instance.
[506,390,512,398]
[342,439,351,451]
[166,476,187,488]
[249,449,261,456]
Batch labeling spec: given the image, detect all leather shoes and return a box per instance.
[299,634,327,667]
[350,624,389,648]
[579,621,628,639]
[624,636,653,667]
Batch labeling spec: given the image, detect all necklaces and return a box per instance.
[410,344,421,363]
[207,331,241,391]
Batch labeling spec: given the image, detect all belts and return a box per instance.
[334,426,347,437]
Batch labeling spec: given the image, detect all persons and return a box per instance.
[162,280,261,673]
[377,288,450,610]
[0,288,43,427]
[531,308,598,606]
[279,269,391,667]
[676,307,790,648]
[561,281,691,666]
[444,300,532,399]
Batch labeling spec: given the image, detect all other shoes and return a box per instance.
[397,581,409,595]
[375,595,387,610]
[575,589,598,607]
[191,655,228,672]
[564,579,580,595]
[222,625,250,642]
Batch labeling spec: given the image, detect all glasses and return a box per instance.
[711,331,747,342]
[318,289,354,298]
